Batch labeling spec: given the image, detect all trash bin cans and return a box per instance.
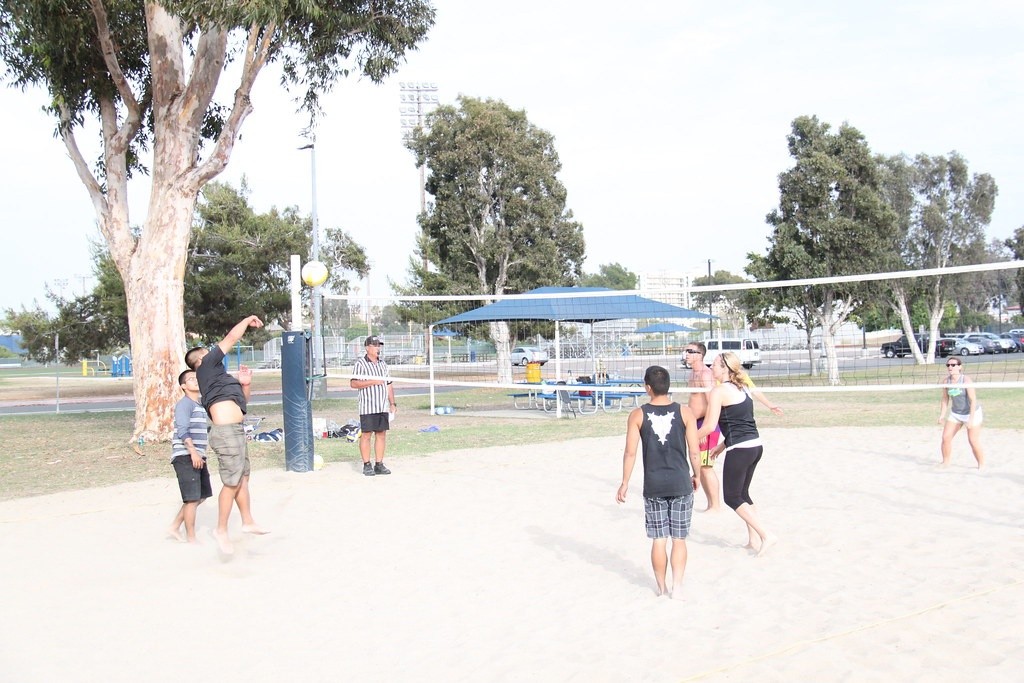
[469,352,476,362]
[591,373,611,405]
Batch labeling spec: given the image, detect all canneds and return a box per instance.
[328,430,331,438]
[323,432,327,438]
[139,437,143,446]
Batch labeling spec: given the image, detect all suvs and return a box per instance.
[962,333,1016,352]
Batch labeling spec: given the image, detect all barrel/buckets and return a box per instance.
[526,363,541,382]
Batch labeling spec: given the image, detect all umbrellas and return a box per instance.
[430,286,720,384]
[633,323,697,345]
[432,326,458,336]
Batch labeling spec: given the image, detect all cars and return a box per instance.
[509,347,548,366]
[937,337,980,356]
[1000,334,1024,350]
[1010,329,1024,334]
[963,338,1001,353]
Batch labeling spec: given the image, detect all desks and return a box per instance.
[605,380,645,388]
[548,384,612,387]
[517,382,556,386]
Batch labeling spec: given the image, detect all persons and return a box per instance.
[615,366,701,599]
[937,357,985,470]
[686,342,720,513]
[165,370,212,546]
[185,315,273,553]
[697,352,782,559]
[350,335,397,475]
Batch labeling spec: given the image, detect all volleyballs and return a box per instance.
[300,259,328,289]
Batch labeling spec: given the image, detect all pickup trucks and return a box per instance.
[880,333,956,357]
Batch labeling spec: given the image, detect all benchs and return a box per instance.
[508,392,642,415]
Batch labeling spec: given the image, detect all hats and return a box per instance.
[364,334,384,346]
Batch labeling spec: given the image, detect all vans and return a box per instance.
[681,339,762,369]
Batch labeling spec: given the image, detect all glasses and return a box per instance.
[686,349,701,354]
[946,364,959,367]
[185,377,198,383]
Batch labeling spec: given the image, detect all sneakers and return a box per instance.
[374,463,391,474]
[363,462,375,476]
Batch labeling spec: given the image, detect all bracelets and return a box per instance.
[391,403,396,406]
